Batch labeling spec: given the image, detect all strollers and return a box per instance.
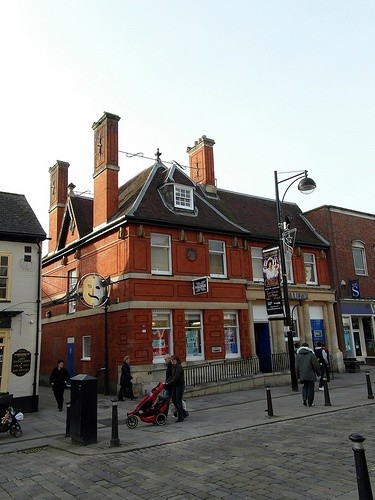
[0,392,22,437]
[126,381,172,429]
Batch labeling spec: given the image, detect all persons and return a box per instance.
[263,260,280,287]
[294,343,321,407]
[192,343,198,356]
[117,355,134,401]
[49,359,69,412]
[162,354,190,423]
[293,343,300,384]
[322,344,330,382]
[313,342,329,391]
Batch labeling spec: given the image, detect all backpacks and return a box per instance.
[314,348,325,367]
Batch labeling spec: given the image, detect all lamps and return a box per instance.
[320,250,327,259]
[180,229,187,241]
[118,226,124,239]
[197,232,205,244]
[242,239,249,251]
[296,246,302,256]
[73,248,82,259]
[61,255,68,265]
[233,236,239,247]
[137,224,145,238]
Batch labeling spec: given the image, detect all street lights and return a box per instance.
[274,169,317,391]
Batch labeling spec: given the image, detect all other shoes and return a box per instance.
[184,413,189,418]
[175,419,184,422]
[309,404,312,407]
[319,387,324,390]
[303,399,307,406]
[118,399,125,401]
[131,397,137,400]
